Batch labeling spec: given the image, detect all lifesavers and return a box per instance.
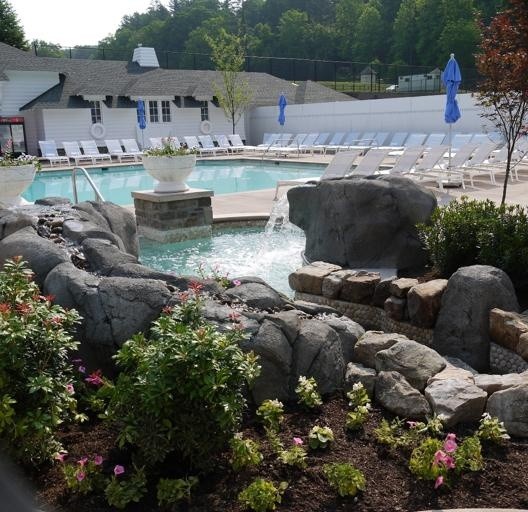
[90,122,106,139]
[200,120,213,135]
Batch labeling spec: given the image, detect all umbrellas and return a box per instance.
[277,93,286,146]
[442,53,463,170]
[135,96,147,152]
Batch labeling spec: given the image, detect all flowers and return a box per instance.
[141,135,198,157]
[0,138,43,173]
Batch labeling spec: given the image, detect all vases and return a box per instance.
[142,157,197,193]
[0,164,37,208]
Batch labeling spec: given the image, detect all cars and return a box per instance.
[385,83,399,93]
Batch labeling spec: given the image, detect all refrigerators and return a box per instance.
[0,116,28,157]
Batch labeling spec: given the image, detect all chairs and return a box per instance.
[254,132,528,201]
[38,133,255,164]
